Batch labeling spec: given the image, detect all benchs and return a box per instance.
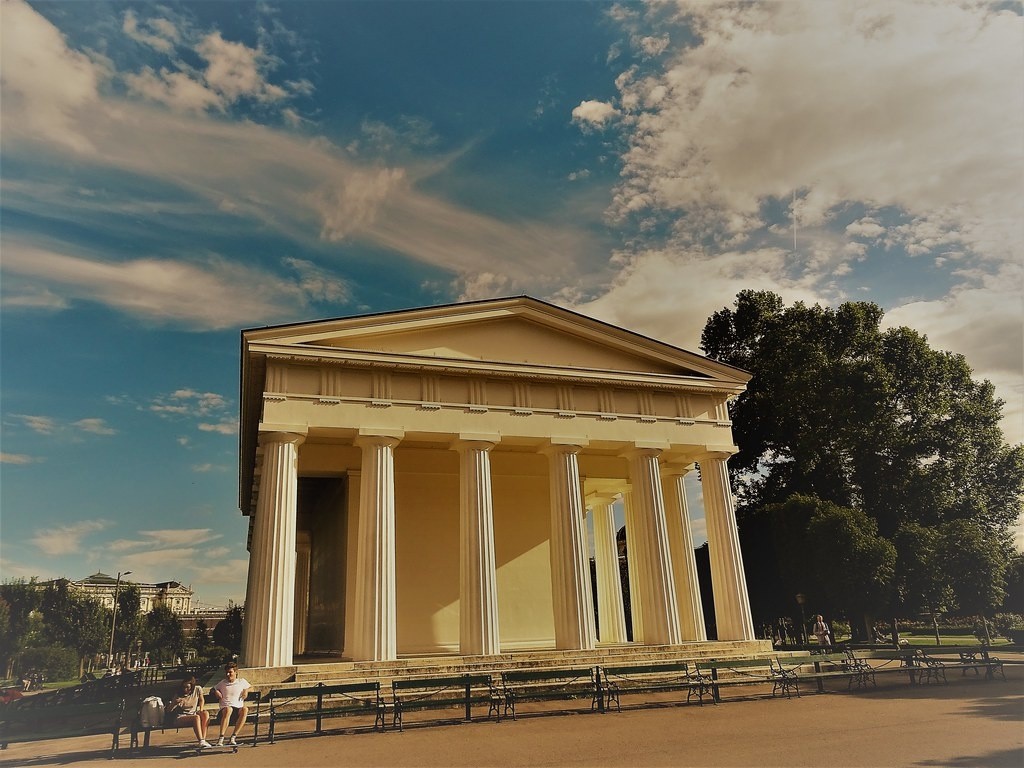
[603,661,704,712]
[0,698,125,761]
[921,647,1006,686]
[391,674,502,733]
[268,681,388,744]
[695,659,792,707]
[501,666,606,721]
[852,649,939,688]
[775,652,868,700]
[129,690,261,752]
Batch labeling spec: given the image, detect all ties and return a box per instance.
[821,624,823,631]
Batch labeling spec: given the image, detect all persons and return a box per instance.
[177,657,181,666]
[169,675,211,747]
[209,662,251,745]
[813,615,831,662]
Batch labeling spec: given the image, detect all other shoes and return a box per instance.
[229,738,236,745]
[199,739,211,748]
[216,737,224,746]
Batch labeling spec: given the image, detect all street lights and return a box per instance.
[105,570,133,677]
[795,592,808,646]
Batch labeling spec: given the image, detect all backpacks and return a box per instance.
[141,696,166,730]
